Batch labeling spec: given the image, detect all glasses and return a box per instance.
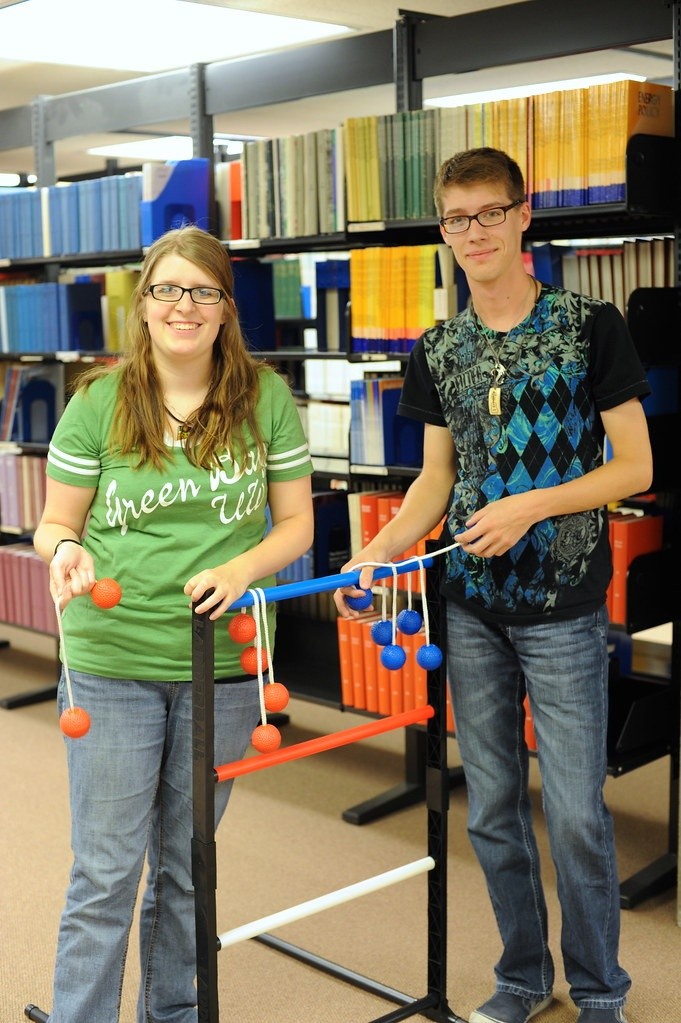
[142,283,225,304]
[439,200,525,233]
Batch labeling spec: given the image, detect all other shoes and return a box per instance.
[575,1005,627,1023]
[469,987,553,1023]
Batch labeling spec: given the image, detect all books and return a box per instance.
[0,269,142,636]
[0,80,675,259]
[562,236,673,323]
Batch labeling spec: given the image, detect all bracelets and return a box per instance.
[53,539,83,556]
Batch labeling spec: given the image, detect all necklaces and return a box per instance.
[163,402,198,441]
[474,274,538,416]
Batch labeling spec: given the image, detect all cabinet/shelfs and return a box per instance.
[1,1,681,913]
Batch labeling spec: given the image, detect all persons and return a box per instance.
[229,244,470,477]
[332,147,653,1023]
[604,505,663,625]
[523,629,634,753]
[602,367,673,493]
[263,489,458,733]
[33,225,315,1023]
